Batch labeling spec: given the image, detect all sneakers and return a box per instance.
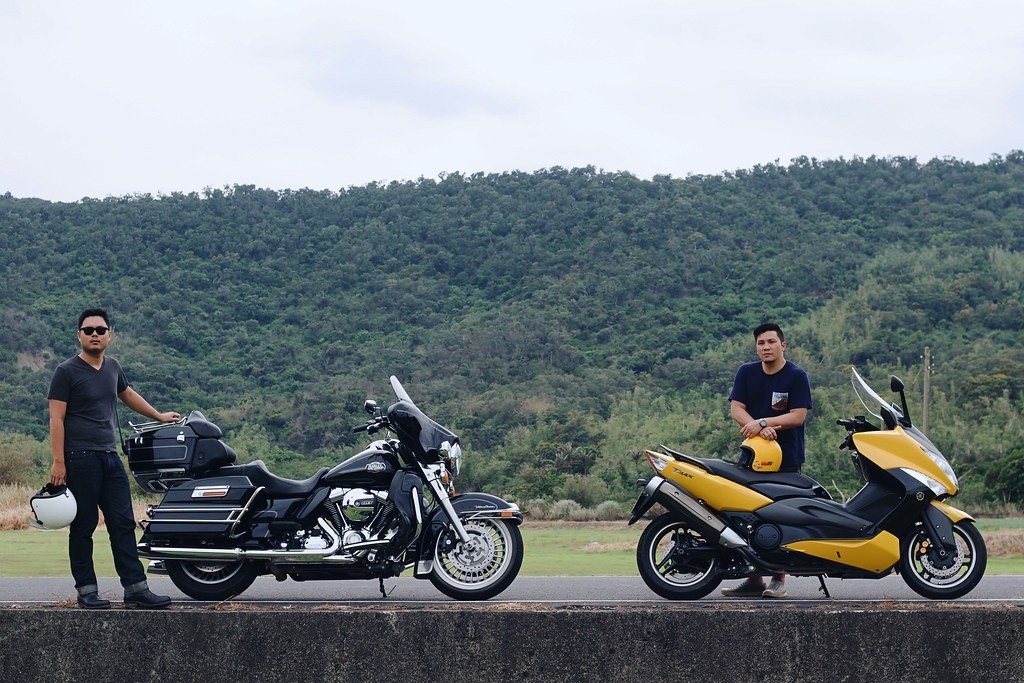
[761,580,787,598]
[721,579,767,597]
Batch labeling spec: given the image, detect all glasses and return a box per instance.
[80,326,110,335]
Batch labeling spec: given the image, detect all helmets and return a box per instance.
[29,481,77,528]
[740,435,783,473]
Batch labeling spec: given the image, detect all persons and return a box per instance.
[47,309,180,609]
[720,323,812,597]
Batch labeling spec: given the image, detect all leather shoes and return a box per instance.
[124,588,172,609]
[77,593,111,609]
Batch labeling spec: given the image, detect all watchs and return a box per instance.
[759,418,768,428]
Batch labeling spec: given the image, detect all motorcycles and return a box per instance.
[627,367,989,599]
[120,374,525,598]
